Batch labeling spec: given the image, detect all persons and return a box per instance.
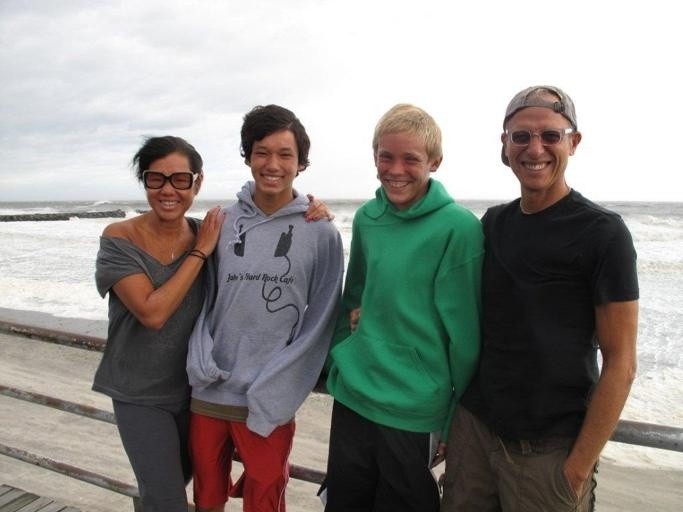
[91,136,335,510]
[436,86,639,511]
[323,104,488,509]
[189,104,345,509]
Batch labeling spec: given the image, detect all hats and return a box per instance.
[501,86,578,167]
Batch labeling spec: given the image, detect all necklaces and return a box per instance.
[167,239,178,262]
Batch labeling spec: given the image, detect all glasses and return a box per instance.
[506,127,573,147]
[143,170,198,190]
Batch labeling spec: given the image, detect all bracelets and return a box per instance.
[189,246,209,261]
